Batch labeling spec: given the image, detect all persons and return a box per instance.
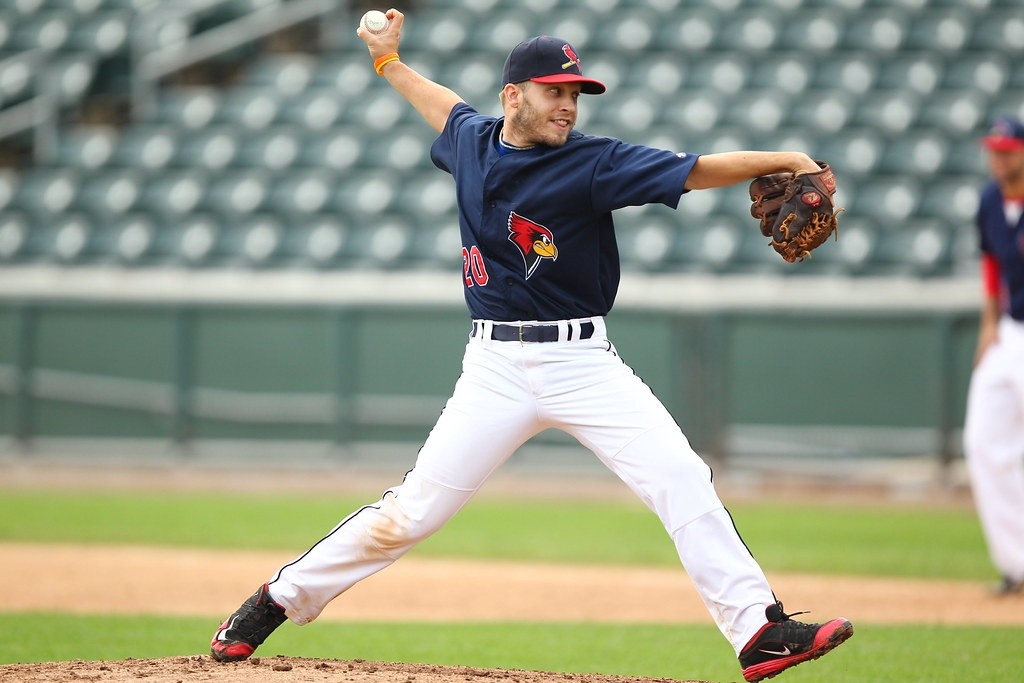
[213,10,854,683]
[963,113,1024,597]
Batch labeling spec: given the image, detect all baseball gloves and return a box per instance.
[749,161,840,262]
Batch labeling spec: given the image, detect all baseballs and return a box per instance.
[358,8,388,33]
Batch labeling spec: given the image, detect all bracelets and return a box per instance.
[375,52,399,76]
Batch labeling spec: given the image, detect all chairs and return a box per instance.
[0,0,1024,286]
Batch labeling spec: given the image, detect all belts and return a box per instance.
[472,323,594,342]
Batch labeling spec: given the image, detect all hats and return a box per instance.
[503,36,606,95]
[981,115,1023,152]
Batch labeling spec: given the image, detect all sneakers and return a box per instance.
[738,601,853,683]
[211,583,288,662]
